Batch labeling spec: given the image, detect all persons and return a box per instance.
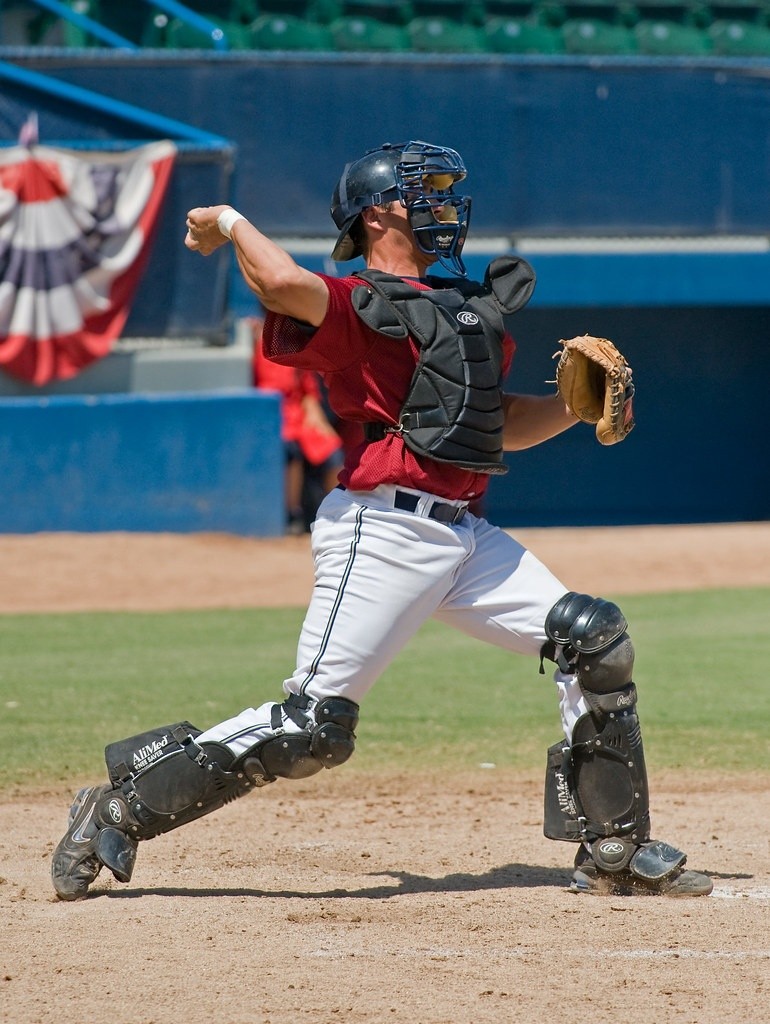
[51,142,712,901]
[247,316,345,534]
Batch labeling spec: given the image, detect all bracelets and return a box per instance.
[217,209,247,240]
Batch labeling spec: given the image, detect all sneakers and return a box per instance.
[51,783,114,900]
[570,842,714,896]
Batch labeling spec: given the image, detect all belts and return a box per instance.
[336,483,469,527]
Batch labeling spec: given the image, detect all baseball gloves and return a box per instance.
[556,335,636,449]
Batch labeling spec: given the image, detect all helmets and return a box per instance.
[331,139,468,263]
